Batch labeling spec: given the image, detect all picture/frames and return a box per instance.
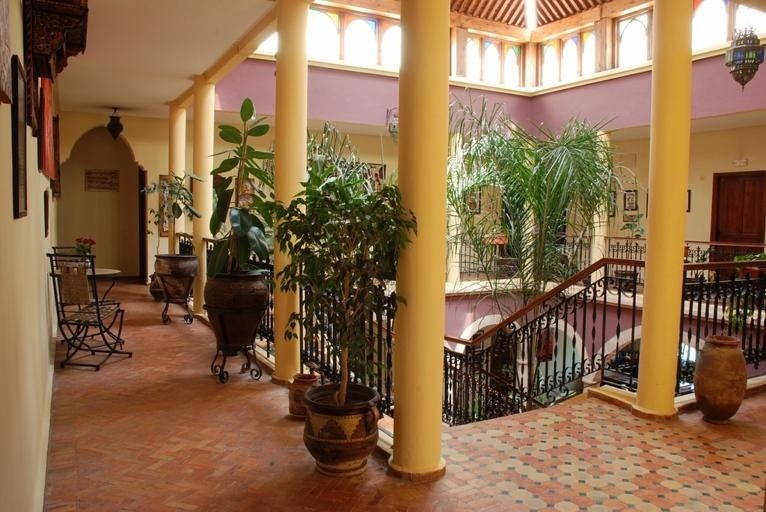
[159,175,170,237]
[11,54,27,218]
[608,191,615,217]
[623,189,637,210]
[463,185,481,214]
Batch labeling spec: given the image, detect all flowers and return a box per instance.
[75,236,97,256]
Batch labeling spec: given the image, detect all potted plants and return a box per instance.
[155,168,207,304]
[614,212,644,292]
[725,253,766,337]
[139,180,167,302]
[685,246,716,301]
[205,96,274,345]
[262,150,419,479]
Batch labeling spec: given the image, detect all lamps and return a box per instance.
[724,2,766,94]
[106,108,123,142]
[385,107,399,144]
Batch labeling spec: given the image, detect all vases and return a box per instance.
[82,252,91,271]
[693,335,747,425]
[289,374,318,419]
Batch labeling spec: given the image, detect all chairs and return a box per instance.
[46,253,132,371]
[53,246,96,310]
